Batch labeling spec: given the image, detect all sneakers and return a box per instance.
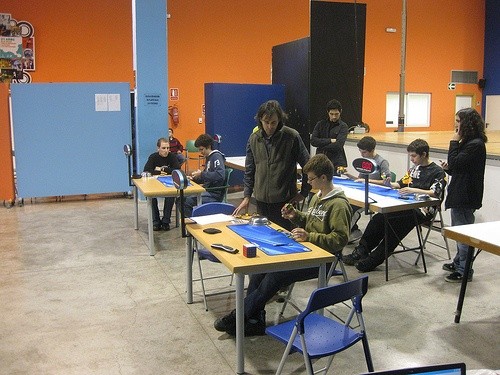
[213,309,237,332]
[227,319,266,336]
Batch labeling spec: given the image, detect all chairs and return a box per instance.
[278,210,361,324]
[184,140,204,173]
[265,273,375,375]
[399,177,452,266]
[193,201,237,311]
[197,168,232,204]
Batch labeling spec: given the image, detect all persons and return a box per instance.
[213,154,353,336]
[175,133,225,218]
[439,108,487,282]
[337,136,391,243]
[231,100,311,302]
[310,99,349,176]
[144,138,181,231]
[169,128,186,166]
[341,138,446,272]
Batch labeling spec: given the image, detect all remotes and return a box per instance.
[211,243,238,253]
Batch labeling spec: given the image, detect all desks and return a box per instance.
[225,156,303,173]
[298,175,439,280]
[441,219,500,322]
[130,174,207,256]
[184,213,337,374]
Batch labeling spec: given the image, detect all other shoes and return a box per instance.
[348,229,362,242]
[274,290,290,303]
[445,271,472,282]
[355,251,381,271]
[342,248,368,266]
[162,224,170,231]
[153,224,161,231]
[442,262,456,272]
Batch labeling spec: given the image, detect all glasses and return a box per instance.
[309,174,322,184]
